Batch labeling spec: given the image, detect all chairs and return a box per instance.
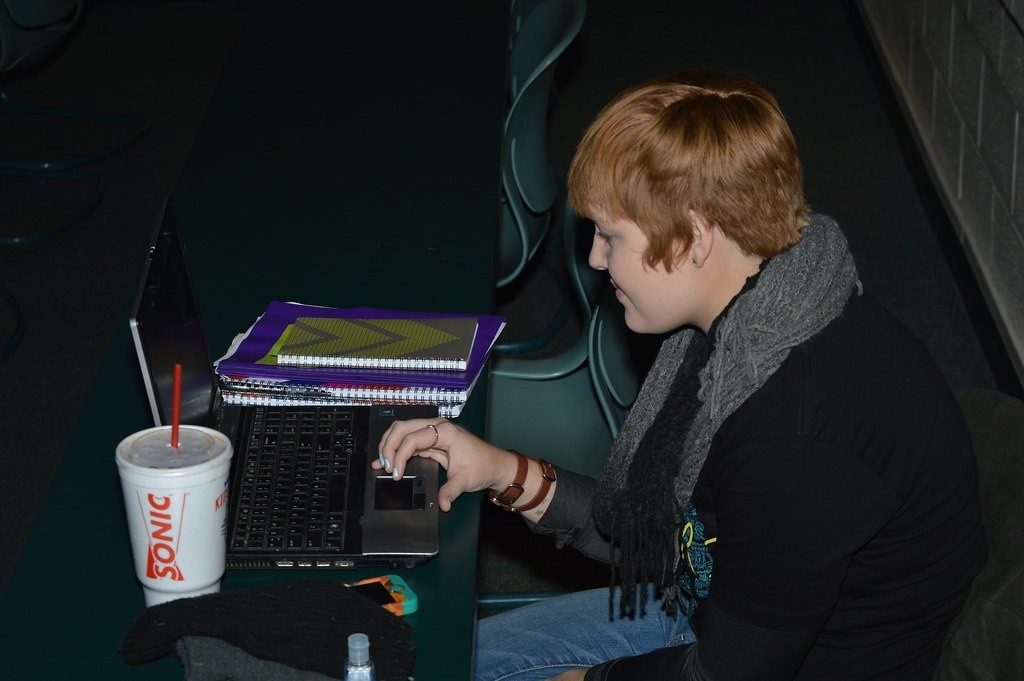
[496,1,587,290]
[486,197,640,524]
[0,0,153,171]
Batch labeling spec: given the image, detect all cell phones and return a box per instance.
[343,575,419,616]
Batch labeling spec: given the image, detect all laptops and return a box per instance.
[131,195,440,570]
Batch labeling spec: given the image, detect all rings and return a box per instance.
[427,425,439,447]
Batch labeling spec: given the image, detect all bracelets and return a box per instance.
[489,449,555,514]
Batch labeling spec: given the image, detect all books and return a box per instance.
[213,299,505,419]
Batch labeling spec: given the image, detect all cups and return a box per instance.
[115,425,235,593]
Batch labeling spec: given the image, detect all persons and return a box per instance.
[370,66,989,681]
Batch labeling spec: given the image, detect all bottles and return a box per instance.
[344,633,376,681]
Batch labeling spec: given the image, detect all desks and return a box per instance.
[0,0,511,680]
